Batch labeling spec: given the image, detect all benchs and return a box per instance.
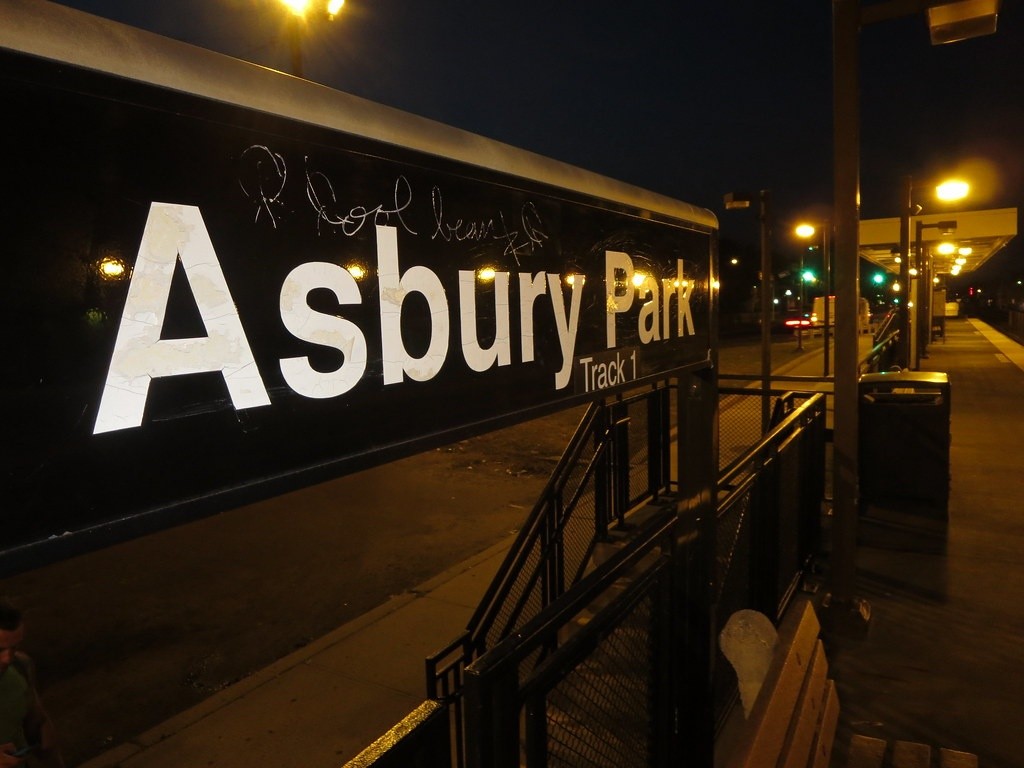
[732,599,977,768]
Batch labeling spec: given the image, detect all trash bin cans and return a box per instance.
[857,371,952,522]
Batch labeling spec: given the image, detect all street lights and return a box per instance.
[908,219,971,372]
[795,222,830,376]
[899,175,970,380]
